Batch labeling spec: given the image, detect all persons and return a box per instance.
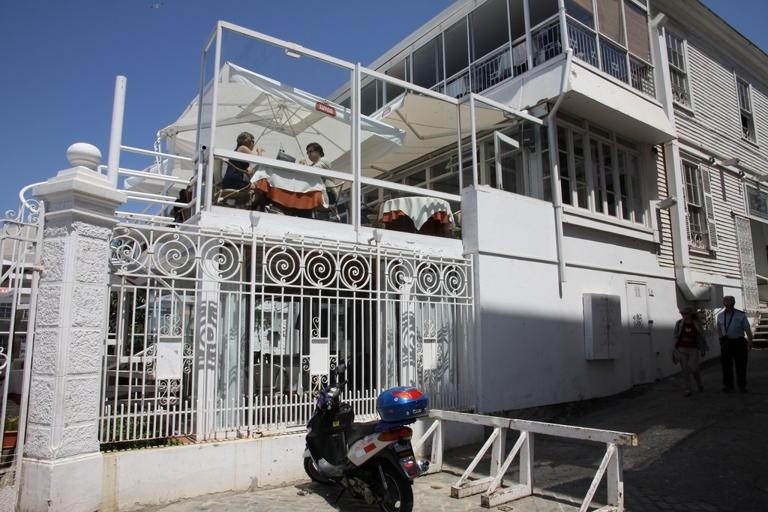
[306,143,337,206]
[174,189,186,212]
[717,296,752,393]
[673,306,705,397]
[223,133,265,211]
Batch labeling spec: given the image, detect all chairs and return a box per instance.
[313,181,346,222]
[211,154,257,209]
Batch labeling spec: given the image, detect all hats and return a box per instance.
[679,307,698,314]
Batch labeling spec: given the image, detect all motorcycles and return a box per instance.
[302,362,428,512]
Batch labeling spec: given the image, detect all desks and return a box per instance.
[380,196,455,237]
[252,165,330,221]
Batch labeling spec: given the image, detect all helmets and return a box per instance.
[319,458,344,477]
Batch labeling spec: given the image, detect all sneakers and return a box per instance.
[683,377,704,397]
[723,385,751,393]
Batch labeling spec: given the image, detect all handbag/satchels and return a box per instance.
[673,348,680,365]
